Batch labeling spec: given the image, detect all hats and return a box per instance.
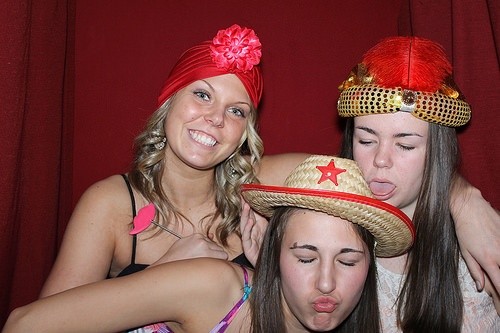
[338,37,471,127]
[239,155,416,258]
[155,24,262,112]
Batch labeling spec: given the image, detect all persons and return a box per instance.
[0,154,416,333]
[38,24,500,333]
[240,36,499,333]
